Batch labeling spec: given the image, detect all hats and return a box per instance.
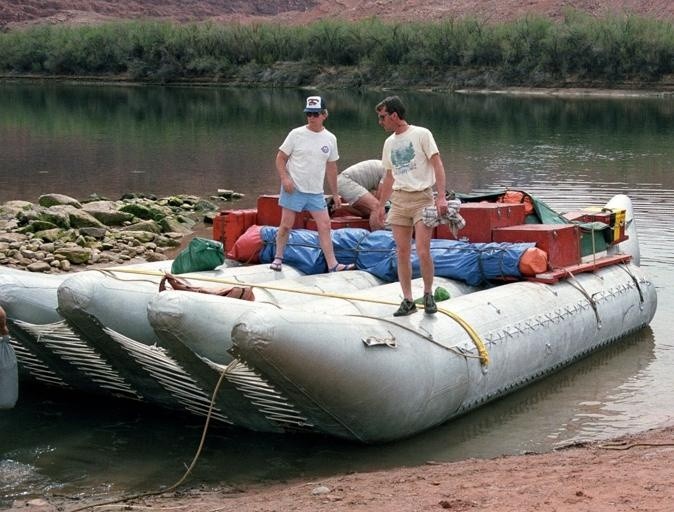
[303,95,327,113]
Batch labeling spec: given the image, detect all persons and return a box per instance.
[270,96,355,272]
[337,157,385,232]
[375,95,448,316]
[0,306,9,336]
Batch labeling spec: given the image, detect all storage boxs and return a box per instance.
[435,199,627,270]
[211,190,371,265]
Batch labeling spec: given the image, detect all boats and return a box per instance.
[0,193,658,445]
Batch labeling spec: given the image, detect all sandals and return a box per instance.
[328,262,356,272]
[270,256,284,272]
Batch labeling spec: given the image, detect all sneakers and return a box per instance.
[423,294,438,314]
[392,298,418,317]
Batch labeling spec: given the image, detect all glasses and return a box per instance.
[378,113,390,121]
[307,112,323,118]
